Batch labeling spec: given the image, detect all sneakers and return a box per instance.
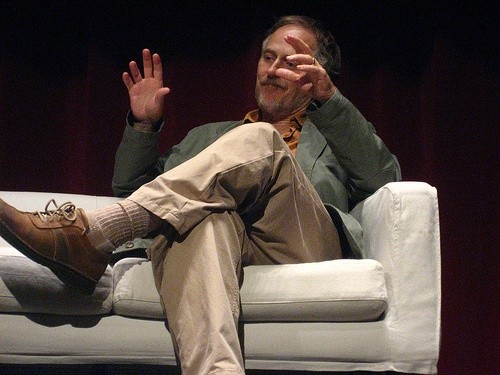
[0,199,111,294]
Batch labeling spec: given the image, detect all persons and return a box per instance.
[0,15,403,374]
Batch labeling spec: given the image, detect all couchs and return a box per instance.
[0,182,439,375]
